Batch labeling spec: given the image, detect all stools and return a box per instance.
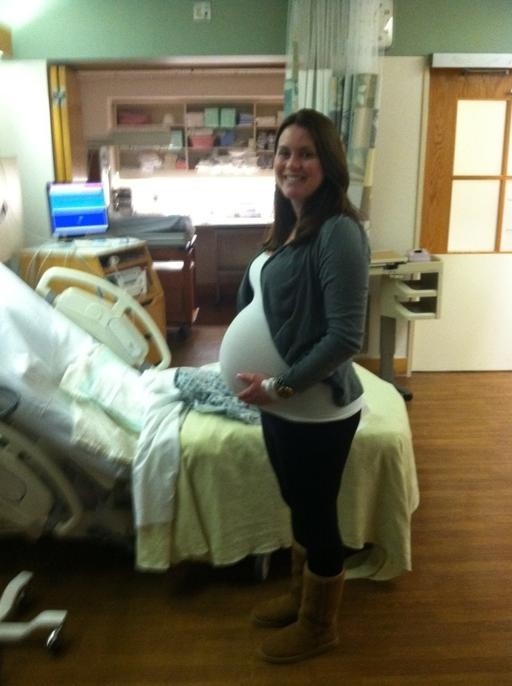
[0,568,70,649]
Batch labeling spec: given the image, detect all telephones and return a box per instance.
[407,247,431,262]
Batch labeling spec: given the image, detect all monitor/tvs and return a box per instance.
[44,181,109,238]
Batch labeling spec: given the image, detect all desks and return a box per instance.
[367,253,444,400]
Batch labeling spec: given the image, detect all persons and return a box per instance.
[218,109,373,666]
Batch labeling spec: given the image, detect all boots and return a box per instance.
[249,540,307,631]
[257,562,346,666]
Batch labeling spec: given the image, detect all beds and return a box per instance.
[1,267,418,582]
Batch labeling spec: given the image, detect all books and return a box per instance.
[370,249,409,264]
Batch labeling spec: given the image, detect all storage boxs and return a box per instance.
[188,135,217,149]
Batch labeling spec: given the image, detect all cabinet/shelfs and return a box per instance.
[20,237,167,367]
[62,234,200,341]
[107,94,285,177]
